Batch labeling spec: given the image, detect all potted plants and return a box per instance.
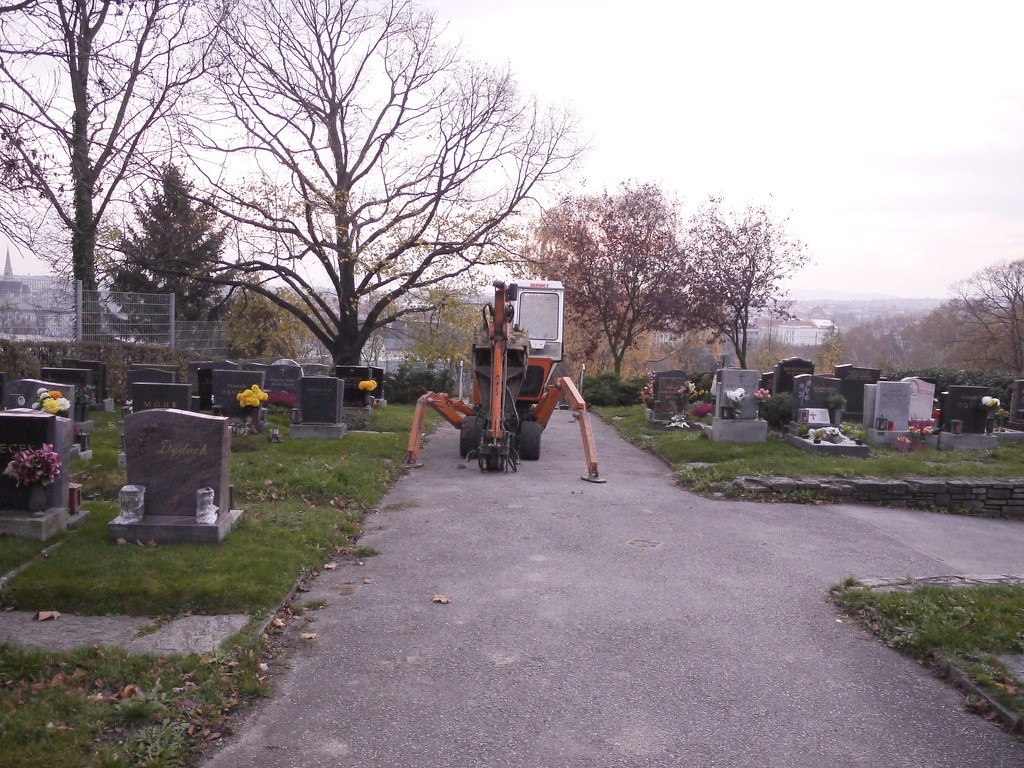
[799,425,810,439]
[811,430,823,444]
[854,431,866,445]
[824,391,846,425]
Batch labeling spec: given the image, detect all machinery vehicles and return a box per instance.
[401,280,607,484]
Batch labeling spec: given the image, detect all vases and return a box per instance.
[997,419,1008,426]
[23,481,46,518]
[243,405,252,421]
[362,390,370,405]
[753,402,765,421]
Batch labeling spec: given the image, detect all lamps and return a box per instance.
[290,406,303,425]
[875,414,888,430]
[719,403,730,420]
[950,419,963,434]
[121,404,132,419]
[212,403,225,416]
[75,429,90,452]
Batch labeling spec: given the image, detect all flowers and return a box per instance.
[978,396,1010,423]
[821,429,835,441]
[373,398,384,408]
[2,443,63,488]
[754,388,772,403]
[677,378,705,405]
[907,413,939,442]
[687,400,715,421]
[726,387,749,410]
[666,414,690,428]
[986,418,993,435]
[640,386,655,411]
[358,379,377,392]
[894,436,913,453]
[26,387,71,418]
[236,384,269,408]
[268,429,284,444]
[994,409,1009,419]
[263,390,297,409]
